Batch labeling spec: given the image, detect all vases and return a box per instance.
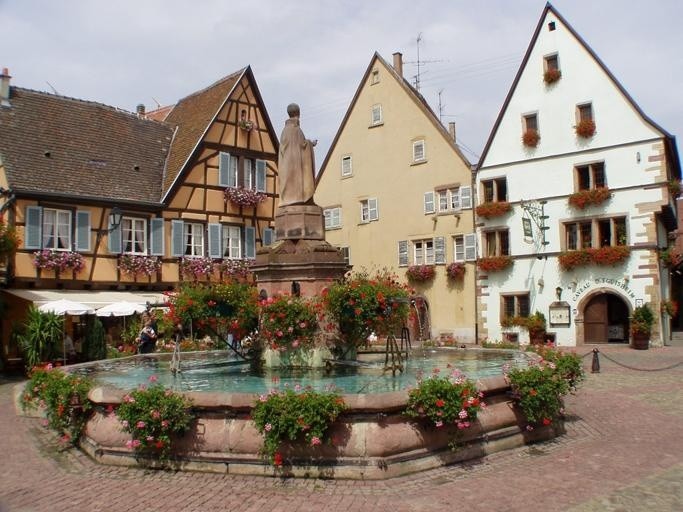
[630,332,650,350]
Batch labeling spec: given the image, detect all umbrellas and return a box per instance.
[35,298,96,365]
[96,302,147,329]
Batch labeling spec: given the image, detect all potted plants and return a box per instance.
[14,300,68,376]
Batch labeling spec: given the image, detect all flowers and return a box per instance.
[588,186,613,205]
[630,304,654,332]
[660,246,681,273]
[406,263,435,284]
[500,362,569,435]
[424,336,584,392]
[182,257,215,277]
[159,279,261,343]
[666,176,681,199]
[476,200,509,219]
[661,299,677,317]
[592,244,629,266]
[402,363,488,437]
[33,251,86,274]
[117,253,163,278]
[318,266,417,360]
[474,255,510,273]
[522,127,540,147]
[504,311,547,336]
[223,186,268,208]
[256,289,319,353]
[119,374,198,458]
[556,248,594,271]
[574,117,595,137]
[222,256,256,279]
[445,261,466,280]
[569,191,588,210]
[1,229,24,262]
[19,370,49,412]
[43,374,99,447]
[251,382,347,466]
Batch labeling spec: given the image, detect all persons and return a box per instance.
[278,103,317,207]
[64,332,73,353]
[75,336,87,355]
[135,310,158,354]
[138,321,156,347]
